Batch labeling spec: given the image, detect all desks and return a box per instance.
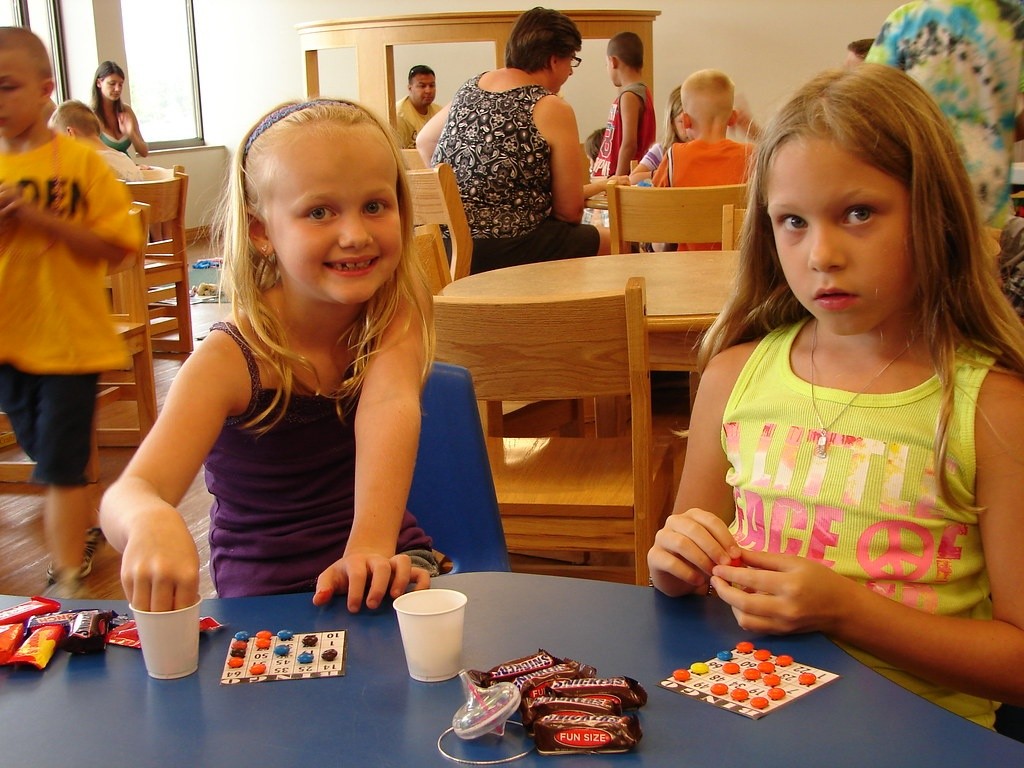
[437,252,740,437]
[0,572,1024,768]
[585,175,608,210]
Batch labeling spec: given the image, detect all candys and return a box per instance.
[0,594,119,671]
[228,628,337,674]
[470,648,648,754]
[673,642,818,710]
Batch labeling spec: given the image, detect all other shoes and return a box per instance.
[653,372,689,390]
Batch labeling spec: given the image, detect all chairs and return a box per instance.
[0,163,190,483]
[398,147,749,582]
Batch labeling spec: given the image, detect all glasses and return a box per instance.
[409,66,433,76]
[569,55,581,68]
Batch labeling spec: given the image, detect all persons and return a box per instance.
[630,86,694,187]
[391,64,443,148]
[97,98,438,609]
[592,31,657,177]
[1,26,143,598]
[862,0,1024,325]
[92,61,148,157]
[47,100,144,181]
[645,63,1024,735]
[416,6,598,275]
[585,128,607,166]
[653,70,755,187]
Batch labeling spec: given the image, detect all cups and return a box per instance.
[393,588,468,683]
[128,592,203,680]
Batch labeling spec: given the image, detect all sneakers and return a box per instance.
[45,505,107,580]
